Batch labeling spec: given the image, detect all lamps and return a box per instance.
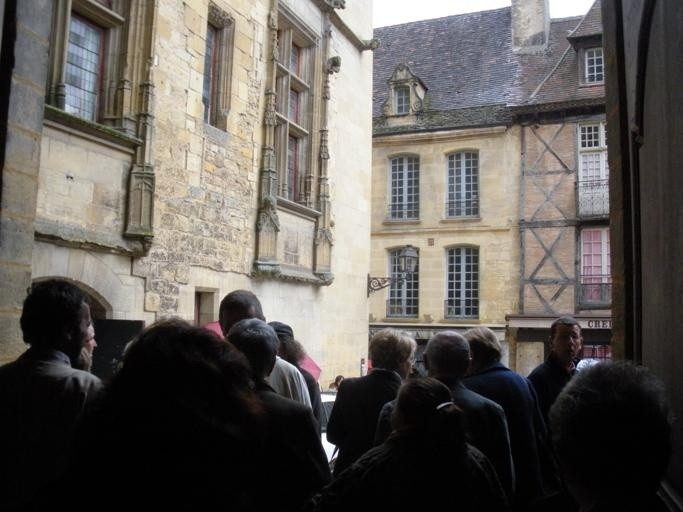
[367,244,419,299]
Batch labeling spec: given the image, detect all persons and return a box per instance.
[0,278,676,511]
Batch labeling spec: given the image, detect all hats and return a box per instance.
[268,322,293,343]
